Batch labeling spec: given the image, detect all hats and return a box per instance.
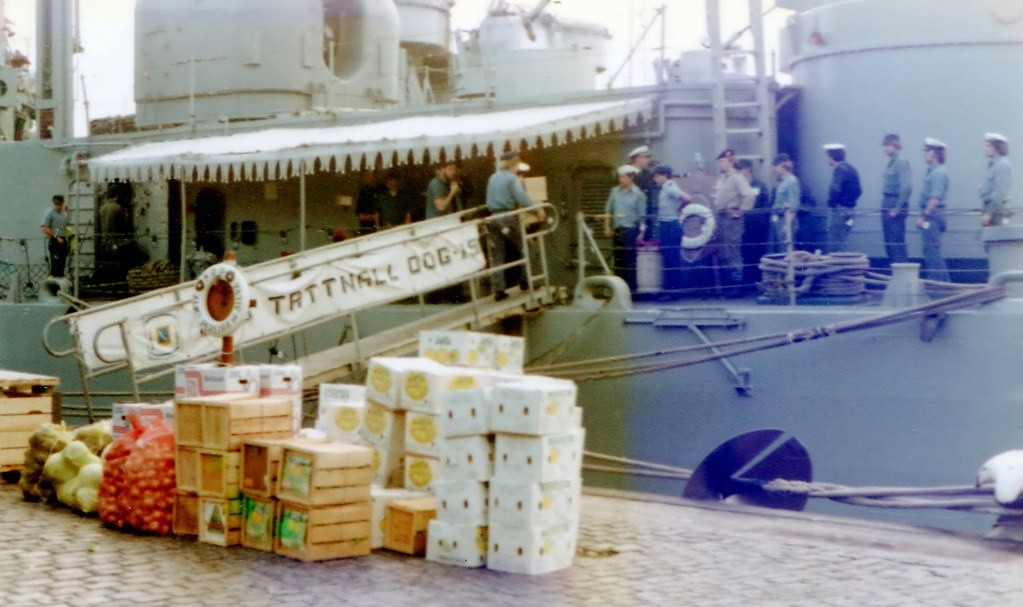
[617,165,634,176]
[628,145,649,159]
[716,148,734,160]
[985,132,1006,145]
[772,153,790,167]
[53,195,64,204]
[502,150,517,160]
[885,135,898,142]
[823,142,846,154]
[922,138,945,152]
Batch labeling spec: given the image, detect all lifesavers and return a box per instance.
[678,202,716,250]
[191,262,251,338]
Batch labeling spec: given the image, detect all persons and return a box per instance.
[882,134,912,263]
[823,143,863,254]
[715,148,815,297]
[100,187,123,242]
[41,195,70,293]
[354,162,473,304]
[604,146,691,301]
[980,133,1011,225]
[485,151,544,302]
[915,137,949,281]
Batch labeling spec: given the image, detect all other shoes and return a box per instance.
[494,290,509,302]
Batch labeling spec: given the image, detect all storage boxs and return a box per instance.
[197,497,241,547]
[489,381,578,435]
[371,490,432,548]
[360,403,407,449]
[365,358,444,411]
[487,523,578,576]
[184,366,260,398]
[404,455,436,493]
[383,499,436,554]
[273,498,375,564]
[171,488,199,535]
[239,438,281,497]
[110,402,149,439]
[487,478,584,530]
[401,367,441,414]
[258,366,303,397]
[195,449,241,499]
[240,494,278,553]
[494,334,524,371]
[438,436,493,482]
[325,401,361,438]
[425,519,489,568]
[493,428,587,481]
[434,481,489,525]
[418,329,497,369]
[406,413,446,458]
[173,444,201,492]
[371,450,405,488]
[439,386,493,438]
[277,442,376,506]
[175,398,202,444]
[134,405,174,434]
[201,396,293,450]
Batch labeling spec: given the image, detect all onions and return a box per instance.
[97,438,175,536]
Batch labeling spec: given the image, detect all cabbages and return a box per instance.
[42,442,104,513]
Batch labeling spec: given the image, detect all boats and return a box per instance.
[0,0,1023,545]
